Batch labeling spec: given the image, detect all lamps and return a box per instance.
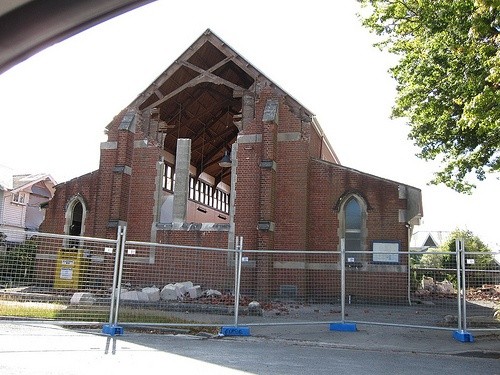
[218,149,232,167]
